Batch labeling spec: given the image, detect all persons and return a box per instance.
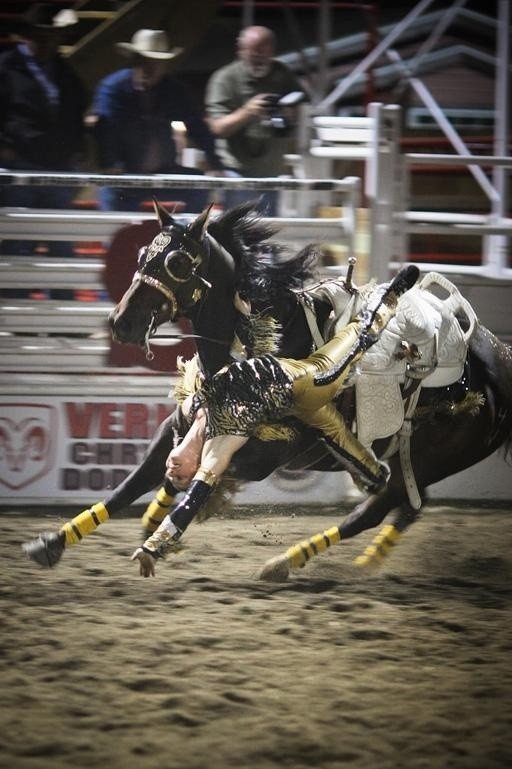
[130,265,421,577]
[205,26,303,218]
[84,28,221,303]
[3,2,94,336]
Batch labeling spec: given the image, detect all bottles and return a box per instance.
[115,30,186,61]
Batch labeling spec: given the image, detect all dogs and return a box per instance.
[18,191,511,584]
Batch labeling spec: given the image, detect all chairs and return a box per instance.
[381,265,419,299]
